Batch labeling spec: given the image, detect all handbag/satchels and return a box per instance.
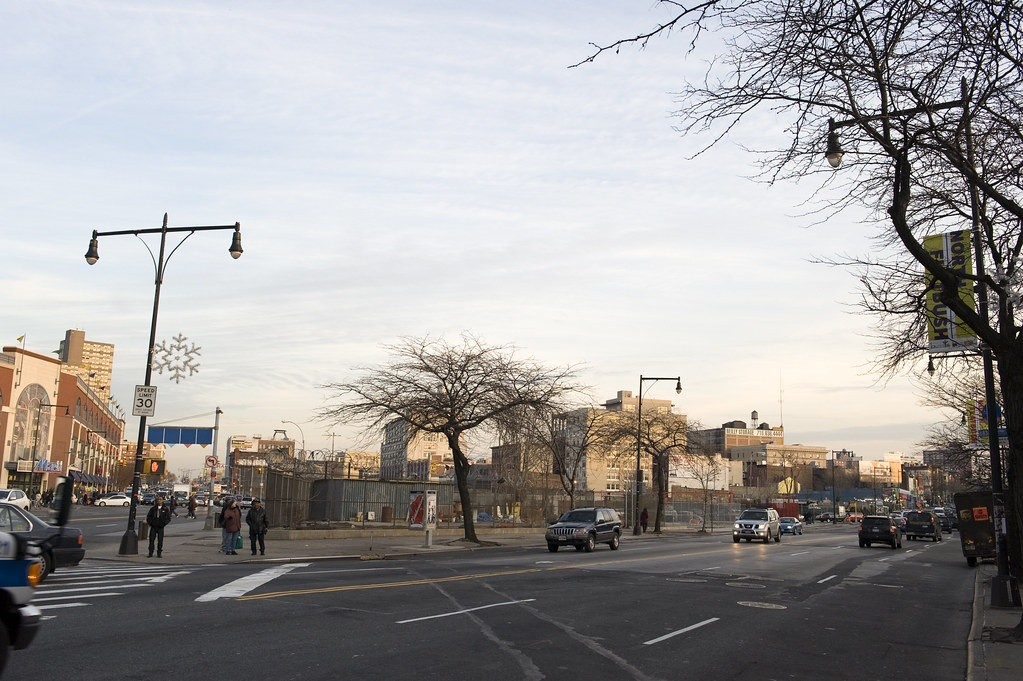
[236,533,243,548]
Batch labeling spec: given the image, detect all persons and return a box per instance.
[83,492,87,506]
[0,588,39,673]
[170,496,179,517]
[219,498,242,555]
[146,496,171,558]
[804,511,812,525]
[641,508,649,533]
[184,495,198,519]
[31,488,55,507]
[202,495,218,506]
[245,497,266,555]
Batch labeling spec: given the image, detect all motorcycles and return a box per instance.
[953,490,998,569]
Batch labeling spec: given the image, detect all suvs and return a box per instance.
[904,509,943,542]
[857,514,903,549]
[731,506,782,544]
[544,505,624,554]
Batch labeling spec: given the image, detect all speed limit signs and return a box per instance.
[132,385,157,417]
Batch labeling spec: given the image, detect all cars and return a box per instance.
[93,483,256,509]
[0,502,87,676]
[807,501,960,535]
[780,516,803,536]
[0,488,32,512]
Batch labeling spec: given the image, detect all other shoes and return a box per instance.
[226,551,230,555]
[231,551,238,555]
[260,551,265,556]
[157,553,162,558]
[252,550,257,555]
[147,554,153,558]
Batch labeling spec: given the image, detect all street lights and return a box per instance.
[281,420,305,453]
[632,374,683,536]
[27,398,70,499]
[82,211,243,557]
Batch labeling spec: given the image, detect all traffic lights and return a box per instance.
[149,459,166,476]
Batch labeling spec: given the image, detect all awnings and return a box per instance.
[70,471,111,484]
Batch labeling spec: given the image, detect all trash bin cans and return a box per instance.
[138,520,148,540]
[214,512,222,528]
